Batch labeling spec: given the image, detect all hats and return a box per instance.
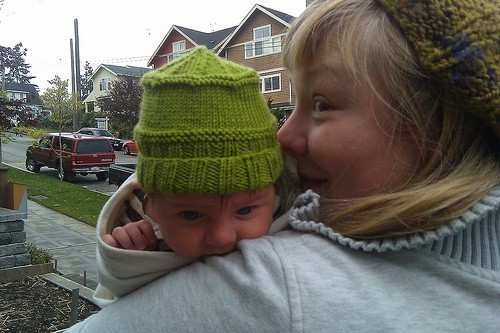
[376,1,499,139]
[133,45,284,196]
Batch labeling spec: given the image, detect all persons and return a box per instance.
[51,0,499,333]
[90,43,284,308]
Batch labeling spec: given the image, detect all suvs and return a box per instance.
[25,132,116,181]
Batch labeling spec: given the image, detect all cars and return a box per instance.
[74,128,123,151]
[122,139,140,155]
[26,118,41,127]
